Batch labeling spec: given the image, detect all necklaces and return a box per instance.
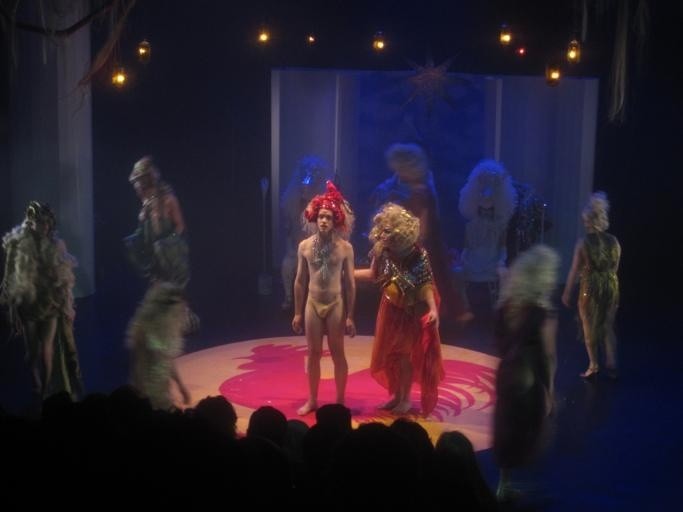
[141,190,158,207]
[312,235,334,282]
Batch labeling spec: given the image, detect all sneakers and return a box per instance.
[579,367,618,382]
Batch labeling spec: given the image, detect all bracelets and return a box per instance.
[170,232,179,244]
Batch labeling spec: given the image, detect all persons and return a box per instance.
[1,384,499,509]
[122,156,194,405]
[454,159,518,330]
[291,181,355,416]
[2,201,74,394]
[369,143,444,317]
[491,245,559,502]
[279,157,336,310]
[560,199,622,378]
[341,204,444,416]
